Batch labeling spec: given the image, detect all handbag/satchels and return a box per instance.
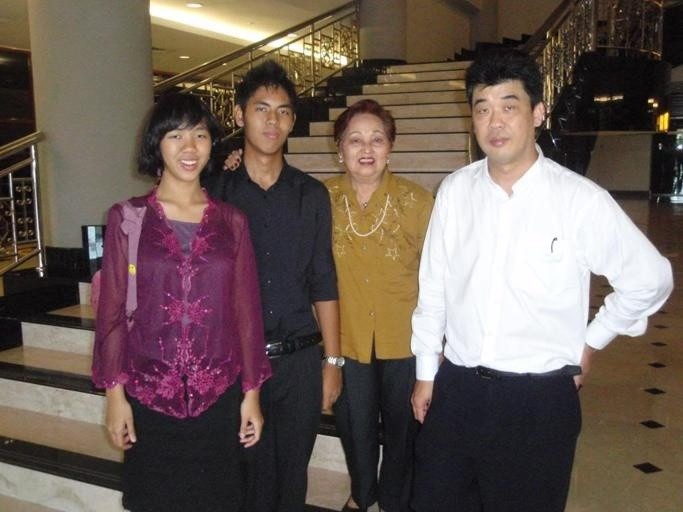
[92,199,147,333]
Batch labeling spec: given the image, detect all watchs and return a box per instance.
[318,353,346,370]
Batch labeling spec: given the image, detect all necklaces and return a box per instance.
[343,194,391,238]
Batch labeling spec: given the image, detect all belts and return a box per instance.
[266,332,322,359]
[476,365,582,381]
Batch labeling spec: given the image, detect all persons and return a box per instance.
[199,57,347,512]
[217,98,439,511]
[407,41,676,511]
[92,85,273,511]
